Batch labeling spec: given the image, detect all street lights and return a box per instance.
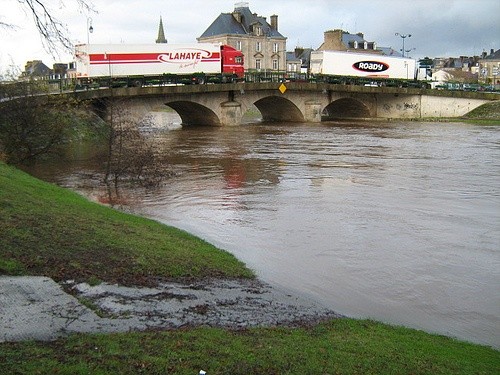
[395,32,411,57]
[399,47,416,58]
[86,17,94,44]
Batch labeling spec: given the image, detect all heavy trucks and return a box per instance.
[308,50,435,90]
[73,42,245,87]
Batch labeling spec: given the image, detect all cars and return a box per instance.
[218,73,309,83]
[436,80,498,93]
